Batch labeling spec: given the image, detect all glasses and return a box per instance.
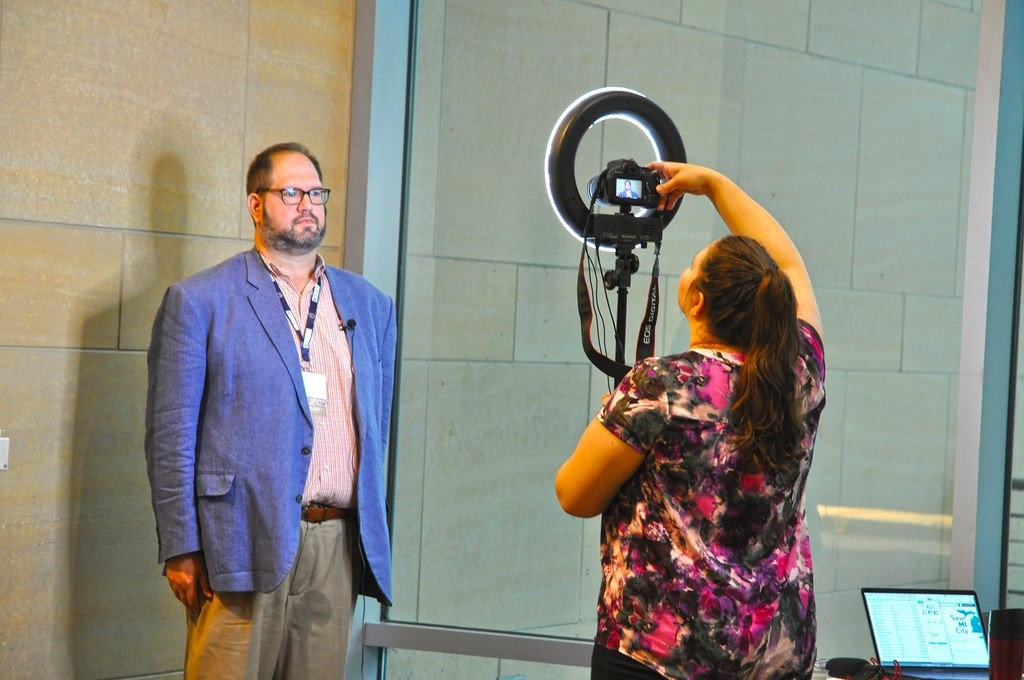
[255,185,330,207]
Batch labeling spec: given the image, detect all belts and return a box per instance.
[298,502,354,522]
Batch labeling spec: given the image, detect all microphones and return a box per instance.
[347,319,357,329]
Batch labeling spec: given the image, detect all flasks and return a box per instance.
[988,607,1024,680]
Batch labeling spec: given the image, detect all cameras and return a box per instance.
[586,159,661,209]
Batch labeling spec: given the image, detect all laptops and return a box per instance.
[862,586,992,680]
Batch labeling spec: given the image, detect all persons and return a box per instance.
[555,161,827,680]
[619,181,639,199]
[145,143,396,680]
